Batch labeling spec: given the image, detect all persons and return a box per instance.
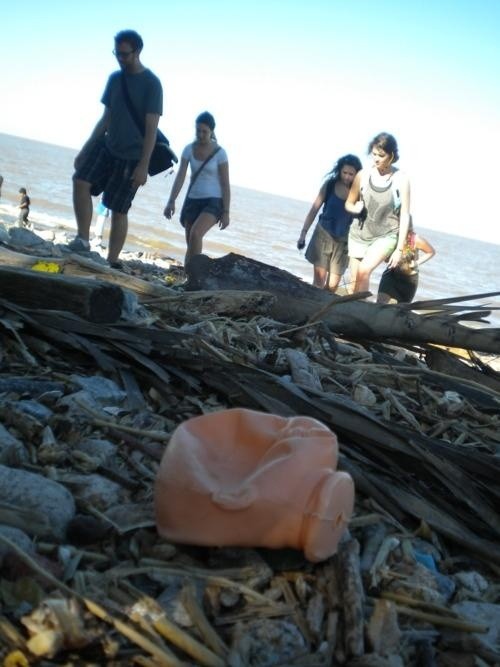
[344,132,411,299]
[91,196,112,246]
[18,188,34,231]
[58,30,162,264]
[298,154,363,293]
[377,216,436,305]
[163,111,230,256]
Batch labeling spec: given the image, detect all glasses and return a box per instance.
[113,49,135,58]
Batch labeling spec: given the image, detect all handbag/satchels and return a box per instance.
[180,197,192,227]
[148,128,178,176]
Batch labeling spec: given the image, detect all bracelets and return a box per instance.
[301,229,308,233]
[219,208,228,214]
[168,198,175,204]
[395,247,403,252]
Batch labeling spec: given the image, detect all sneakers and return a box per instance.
[56,239,90,256]
